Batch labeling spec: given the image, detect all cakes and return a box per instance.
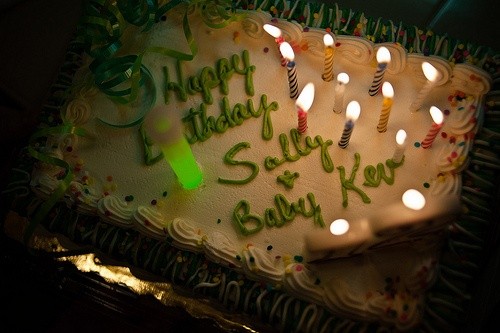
[0,1,500,333]
